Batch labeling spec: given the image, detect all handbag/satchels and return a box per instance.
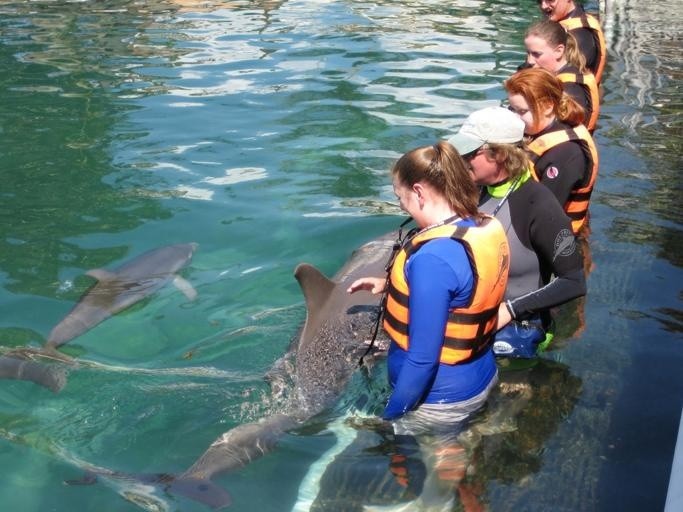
[492,321,546,360]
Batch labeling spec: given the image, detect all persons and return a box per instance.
[444,104,589,491]
[517,21,600,138]
[497,71,598,234]
[346,141,511,511]
[535,0,608,86]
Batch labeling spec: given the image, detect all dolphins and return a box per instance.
[107,224,418,510]
[9,238,200,367]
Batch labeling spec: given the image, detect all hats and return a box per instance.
[447,106,527,156]
